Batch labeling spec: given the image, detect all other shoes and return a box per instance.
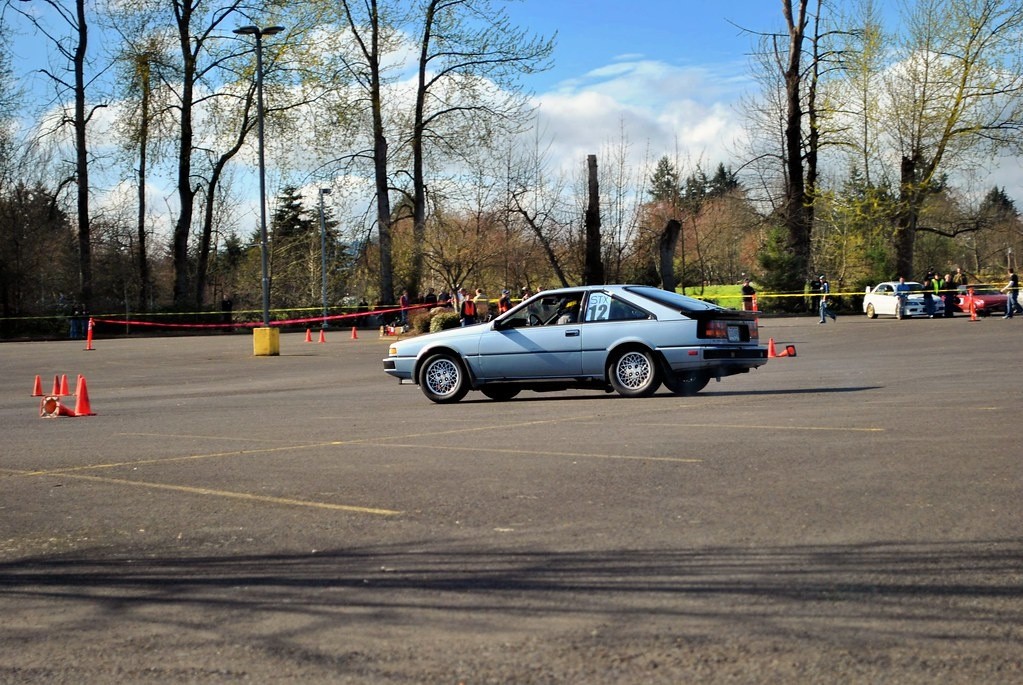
[1002,315,1011,319]
[819,321,825,323]
[834,316,836,323]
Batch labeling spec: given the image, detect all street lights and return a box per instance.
[234,25,286,327]
[319,188,331,326]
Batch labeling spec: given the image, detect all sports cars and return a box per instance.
[939,284,1009,314]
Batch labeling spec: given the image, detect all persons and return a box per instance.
[1000,267,1023,319]
[922,267,968,319]
[69,302,90,340]
[539,293,581,326]
[521,286,549,318]
[220,294,233,332]
[896,276,911,320]
[741,278,755,311]
[342,297,389,330]
[497,290,513,316]
[808,273,837,324]
[400,287,489,327]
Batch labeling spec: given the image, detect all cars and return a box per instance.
[863,282,946,320]
[382,284,769,403]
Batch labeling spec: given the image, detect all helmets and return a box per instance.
[556,297,578,317]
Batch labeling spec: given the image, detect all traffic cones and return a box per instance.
[304,329,311,341]
[39,393,75,416]
[766,338,776,358]
[351,327,358,339]
[74,375,100,417]
[317,330,325,343]
[52,374,70,395]
[31,374,44,396]
[778,345,796,357]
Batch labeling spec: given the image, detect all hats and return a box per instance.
[522,287,529,291]
[503,290,510,294]
[819,276,825,280]
[745,279,749,283]
